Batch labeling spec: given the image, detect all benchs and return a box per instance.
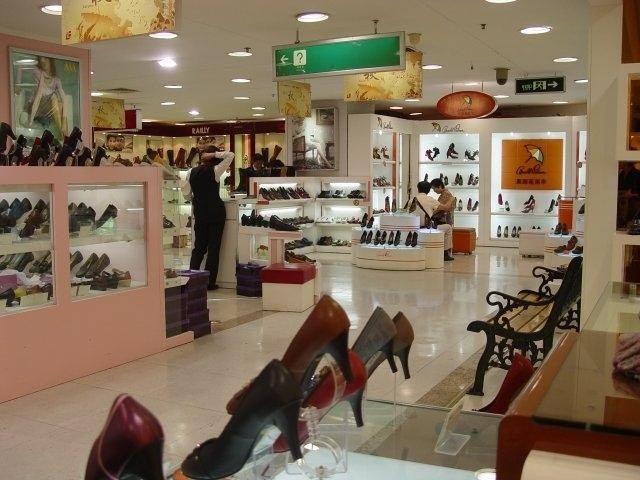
[460,253,580,399]
[517,226,552,259]
[453,226,477,254]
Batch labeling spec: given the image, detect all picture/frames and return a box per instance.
[624,72,640,153]
[6,45,82,156]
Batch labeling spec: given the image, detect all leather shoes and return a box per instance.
[242,187,317,263]
[474,352,535,414]
[361,196,438,247]
[373,177,391,186]
[497,226,521,238]
[317,187,364,246]
[425,143,479,211]
[0,197,131,307]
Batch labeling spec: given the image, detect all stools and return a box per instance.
[258,262,315,315]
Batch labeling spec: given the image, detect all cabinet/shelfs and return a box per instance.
[355,211,439,271]
[493,186,563,240]
[321,179,365,251]
[419,133,480,240]
[94,128,321,275]
[544,239,587,270]
[370,129,398,214]
[0,166,195,406]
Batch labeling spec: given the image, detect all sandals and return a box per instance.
[185,216,192,227]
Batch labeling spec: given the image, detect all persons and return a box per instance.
[181,146,235,291]
[30,58,69,140]
[245,154,265,176]
[431,178,453,225]
[106,134,115,150]
[196,137,205,150]
[408,181,454,261]
[115,135,125,151]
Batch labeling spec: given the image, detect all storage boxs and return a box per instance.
[235,263,263,298]
[157,268,214,342]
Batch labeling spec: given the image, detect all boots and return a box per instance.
[232,145,295,194]
[135,147,197,169]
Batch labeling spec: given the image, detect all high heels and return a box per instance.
[84,393,164,480]
[373,146,381,159]
[273,349,369,453]
[498,193,561,213]
[365,312,414,379]
[381,146,389,158]
[227,295,355,415]
[181,359,303,480]
[0,121,134,167]
[351,306,398,373]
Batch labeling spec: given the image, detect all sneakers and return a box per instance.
[554,223,583,254]
[168,199,179,203]
[444,255,454,261]
